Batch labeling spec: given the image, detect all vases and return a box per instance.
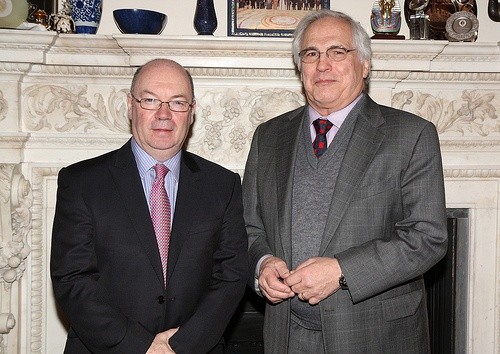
[370,0,405,40]
[67,0,103,35]
[193,0,218,36]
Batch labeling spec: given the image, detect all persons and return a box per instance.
[241,9,448,354]
[284,0,322,8]
[50,59,249,354]
[239,0,281,10]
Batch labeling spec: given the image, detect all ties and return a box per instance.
[311,119,333,158]
[149,165,173,289]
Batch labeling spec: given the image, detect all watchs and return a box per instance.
[339,273,348,290]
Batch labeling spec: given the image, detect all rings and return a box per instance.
[301,292,306,301]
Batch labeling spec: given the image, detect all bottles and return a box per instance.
[66,0,102,34]
[194,0,217,35]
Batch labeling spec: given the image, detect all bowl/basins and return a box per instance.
[113,8,167,35]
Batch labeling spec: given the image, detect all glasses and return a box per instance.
[131,94,194,112]
[298,46,356,63]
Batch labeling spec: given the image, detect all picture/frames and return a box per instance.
[227,0,330,38]
